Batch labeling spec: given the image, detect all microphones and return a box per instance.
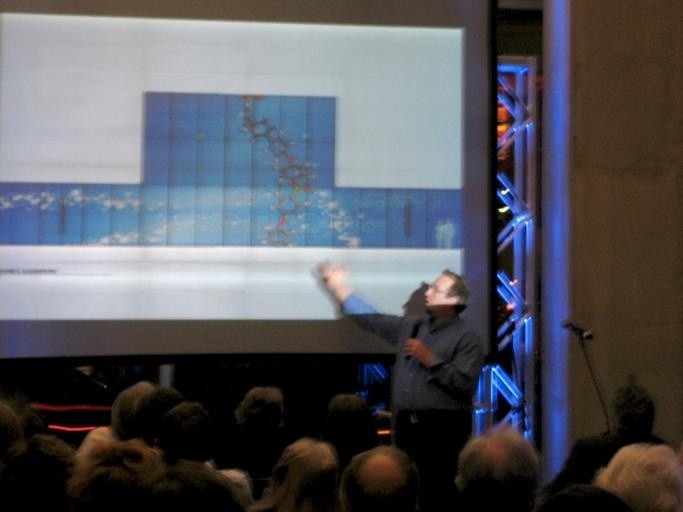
[561,315,594,344]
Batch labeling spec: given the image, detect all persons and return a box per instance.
[322,263,484,512]
[1,381,682,511]
